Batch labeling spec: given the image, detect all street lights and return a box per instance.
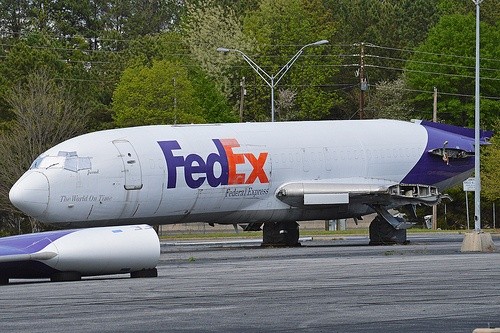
[216,40,330,121]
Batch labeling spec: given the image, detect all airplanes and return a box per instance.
[9,118,496,244]
